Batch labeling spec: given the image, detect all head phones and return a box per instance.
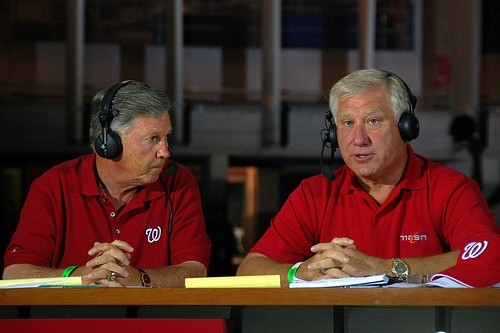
[324,70,419,141]
[94,79,134,160]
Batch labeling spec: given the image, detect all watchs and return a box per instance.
[391,257,410,283]
[138,268,151,288]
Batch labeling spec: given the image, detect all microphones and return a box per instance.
[165,150,178,176]
[320,143,335,181]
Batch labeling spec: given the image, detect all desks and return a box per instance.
[0,258,500,333]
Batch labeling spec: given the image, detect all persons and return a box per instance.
[236,67,500,288]
[2,80,213,287]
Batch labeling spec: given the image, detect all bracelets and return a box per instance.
[287,261,303,284]
[62,265,79,277]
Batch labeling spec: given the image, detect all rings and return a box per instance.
[320,268,326,274]
[106,271,118,280]
